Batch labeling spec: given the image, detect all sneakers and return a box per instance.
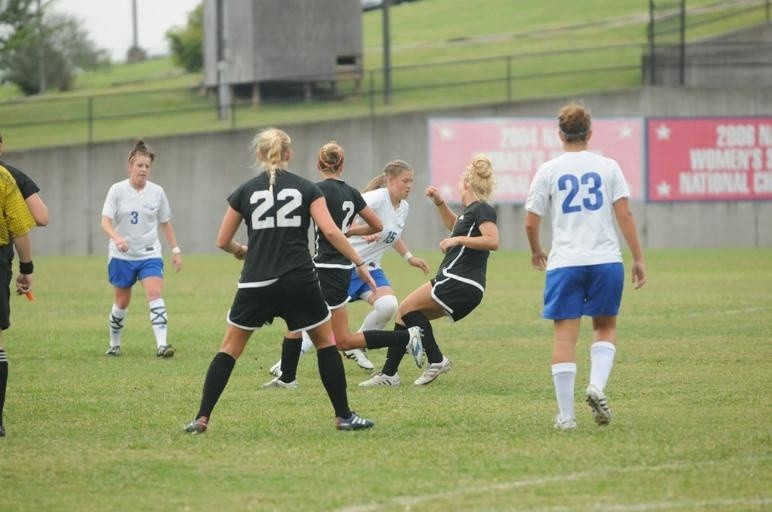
[345,329,450,387]
[105,345,120,357]
[553,416,576,430]
[155,344,176,358]
[264,360,298,388]
[584,386,612,427]
[334,411,374,431]
[183,417,209,434]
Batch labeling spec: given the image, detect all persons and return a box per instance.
[356,157,498,388]
[525,104,647,431]
[261,140,425,390]
[100,138,182,359]
[0,126,47,227]
[0,162,36,436]
[269,159,429,376]
[182,127,378,434]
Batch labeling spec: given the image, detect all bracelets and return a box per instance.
[435,198,445,208]
[18,260,35,274]
[404,251,413,260]
[355,260,368,268]
[171,246,182,252]
[234,246,243,256]
